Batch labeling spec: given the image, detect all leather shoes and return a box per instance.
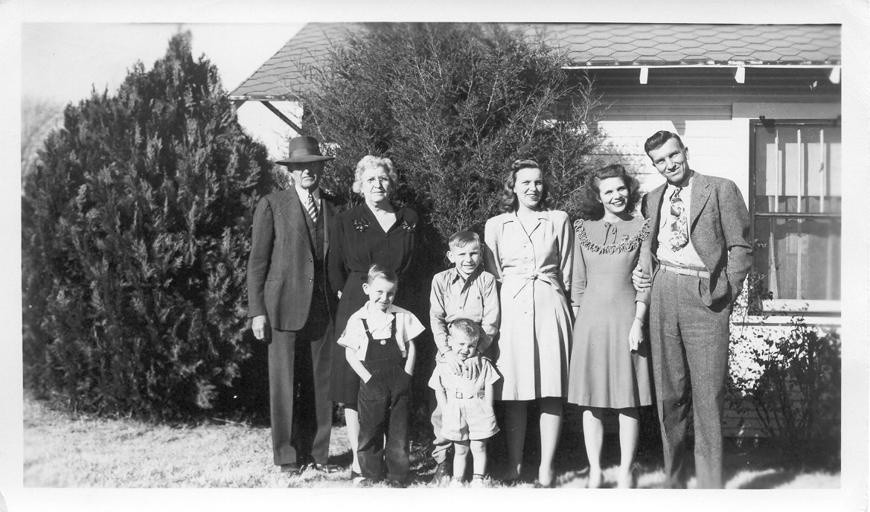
[305,458,343,472]
[281,463,298,478]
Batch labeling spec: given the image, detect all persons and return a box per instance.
[336,263,427,488]
[427,231,501,486]
[632,130,756,489]
[247,136,345,474]
[570,164,653,489]
[484,159,574,489]
[329,155,423,481]
[427,319,500,486]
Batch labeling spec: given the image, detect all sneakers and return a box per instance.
[426,459,451,487]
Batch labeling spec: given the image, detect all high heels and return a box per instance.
[535,460,556,488]
[501,455,524,486]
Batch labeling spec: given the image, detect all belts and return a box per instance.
[660,264,709,279]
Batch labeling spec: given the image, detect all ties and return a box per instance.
[669,187,688,253]
[307,195,318,225]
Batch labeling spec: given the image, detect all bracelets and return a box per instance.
[635,315,646,326]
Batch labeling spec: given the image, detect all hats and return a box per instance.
[275,136,335,165]
